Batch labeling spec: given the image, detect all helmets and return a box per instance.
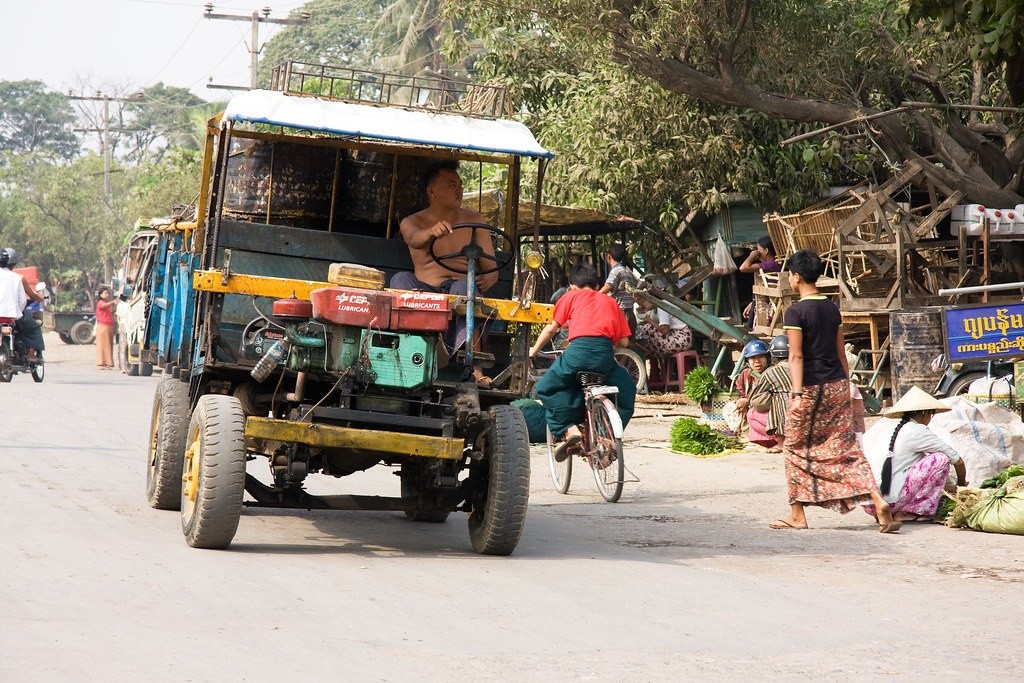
[770,335,790,358]
[744,340,771,358]
[0,249,9,261]
[5,247,18,264]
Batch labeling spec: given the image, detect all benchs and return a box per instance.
[211,219,513,372]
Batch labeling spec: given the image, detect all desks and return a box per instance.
[752,160,1024,403]
[638,216,715,298]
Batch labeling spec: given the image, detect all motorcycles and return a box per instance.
[0,282,50,382]
[931,303,1024,399]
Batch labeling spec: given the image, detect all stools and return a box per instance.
[664,352,699,393]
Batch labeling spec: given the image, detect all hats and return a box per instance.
[883,385,953,419]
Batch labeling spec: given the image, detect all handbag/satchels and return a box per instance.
[712,230,737,275]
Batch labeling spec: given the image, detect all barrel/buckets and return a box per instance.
[888,306,950,406]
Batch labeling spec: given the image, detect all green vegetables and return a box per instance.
[682,365,722,401]
[998,464,1024,479]
[669,417,742,454]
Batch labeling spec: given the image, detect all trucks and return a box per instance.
[137,70,557,558]
[42,309,96,346]
[112,215,161,377]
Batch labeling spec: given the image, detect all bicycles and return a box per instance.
[528,346,641,504]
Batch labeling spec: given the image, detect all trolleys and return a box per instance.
[632,283,889,415]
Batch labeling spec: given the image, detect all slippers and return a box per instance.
[555,434,582,462]
[769,519,808,529]
[900,514,935,525]
[878,522,902,533]
[474,377,492,390]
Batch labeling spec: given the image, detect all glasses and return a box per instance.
[930,413,934,418]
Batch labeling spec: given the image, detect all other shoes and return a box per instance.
[28,357,44,362]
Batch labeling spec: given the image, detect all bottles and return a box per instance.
[251,335,290,383]
[1000,204,1024,234]
[950,204,985,236]
[985,209,1003,235]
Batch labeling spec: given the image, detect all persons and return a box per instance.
[740,236,781,319]
[598,243,638,338]
[549,276,568,304]
[733,340,777,448]
[96,288,117,370]
[390,159,498,389]
[633,293,692,354]
[769,252,903,533]
[750,334,792,454]
[0,246,45,363]
[859,385,969,523]
[528,263,637,462]
